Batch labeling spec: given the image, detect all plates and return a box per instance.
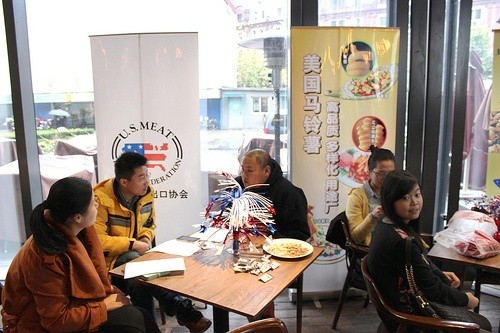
[263,239,313,258]
[346,64,399,100]
[313,225,346,265]
[335,148,371,189]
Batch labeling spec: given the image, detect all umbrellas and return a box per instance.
[47,110,72,117]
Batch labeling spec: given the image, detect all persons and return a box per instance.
[225,150,310,323]
[344,145,429,287]
[83,152,214,333]
[55,115,66,129]
[363,169,493,333]
[1,177,154,333]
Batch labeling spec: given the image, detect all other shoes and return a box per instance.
[177,314,212,333]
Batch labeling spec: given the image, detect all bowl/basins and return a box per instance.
[352,116,387,152]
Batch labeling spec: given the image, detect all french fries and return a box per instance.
[352,154,370,174]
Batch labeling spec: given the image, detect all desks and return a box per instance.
[426,242,500,313]
[108,227,325,333]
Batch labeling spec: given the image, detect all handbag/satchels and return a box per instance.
[434,220,500,258]
[402,235,436,317]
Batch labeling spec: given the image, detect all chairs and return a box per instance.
[331,220,369,329]
[361,255,480,333]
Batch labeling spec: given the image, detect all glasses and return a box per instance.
[372,172,386,178]
[128,172,152,183]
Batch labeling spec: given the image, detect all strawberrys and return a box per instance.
[339,149,354,166]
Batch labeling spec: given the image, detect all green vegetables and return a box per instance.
[351,77,376,96]
